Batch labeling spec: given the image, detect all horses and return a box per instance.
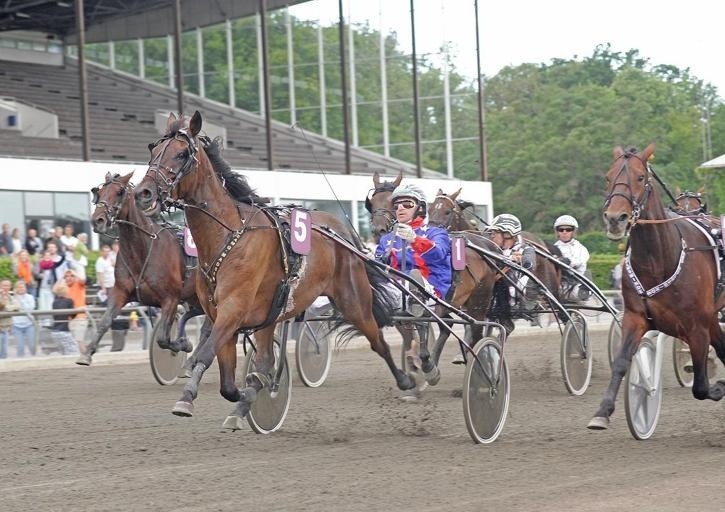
[425,187,564,396]
[585,139,724,433]
[74,169,218,378]
[366,167,484,401]
[132,109,418,432]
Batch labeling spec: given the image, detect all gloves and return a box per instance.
[396,224,416,243]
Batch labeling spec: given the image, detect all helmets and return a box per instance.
[491,214,521,237]
[553,215,578,235]
[391,185,426,201]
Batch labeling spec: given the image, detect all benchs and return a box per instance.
[0,62,388,179]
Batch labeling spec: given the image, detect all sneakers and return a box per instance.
[409,269,425,317]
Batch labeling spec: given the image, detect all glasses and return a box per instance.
[558,228,572,232]
[392,200,414,209]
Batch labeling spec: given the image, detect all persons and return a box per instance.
[551,215,591,301]
[0,224,160,357]
[613,256,626,291]
[312,185,453,321]
[486,214,537,305]
